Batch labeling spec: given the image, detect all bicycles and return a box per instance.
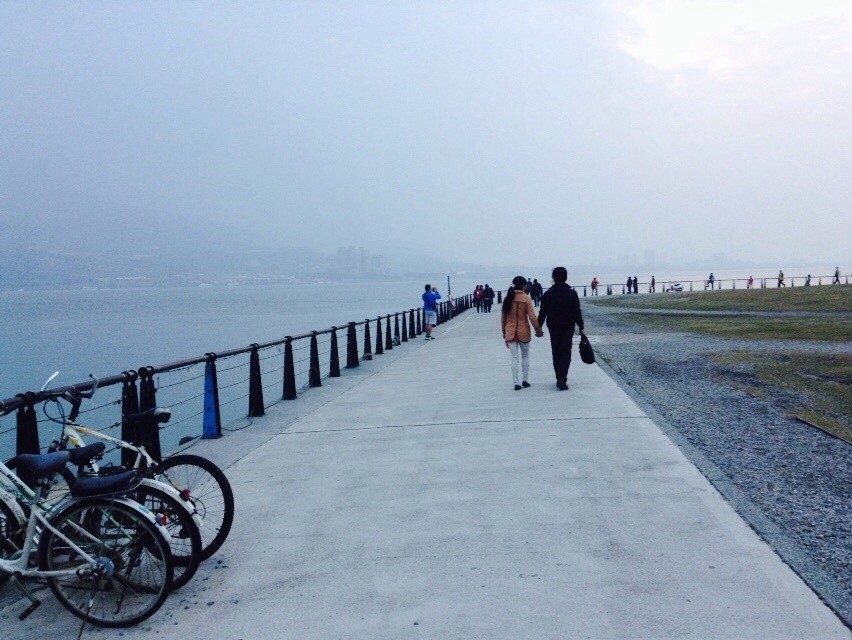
[0,373,234,640]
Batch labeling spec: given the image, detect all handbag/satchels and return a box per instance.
[578,333,595,364]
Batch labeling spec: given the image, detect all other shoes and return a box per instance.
[425,335,435,340]
[514,385,521,390]
[557,382,569,390]
[522,381,530,387]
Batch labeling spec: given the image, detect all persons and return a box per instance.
[627,276,638,294]
[705,273,714,290]
[749,276,753,288]
[501,276,544,390]
[591,277,599,296]
[651,276,655,293]
[835,266,841,285]
[778,270,785,288]
[525,278,543,308]
[805,275,811,286]
[422,284,441,340]
[538,267,585,389]
[473,284,495,313]
